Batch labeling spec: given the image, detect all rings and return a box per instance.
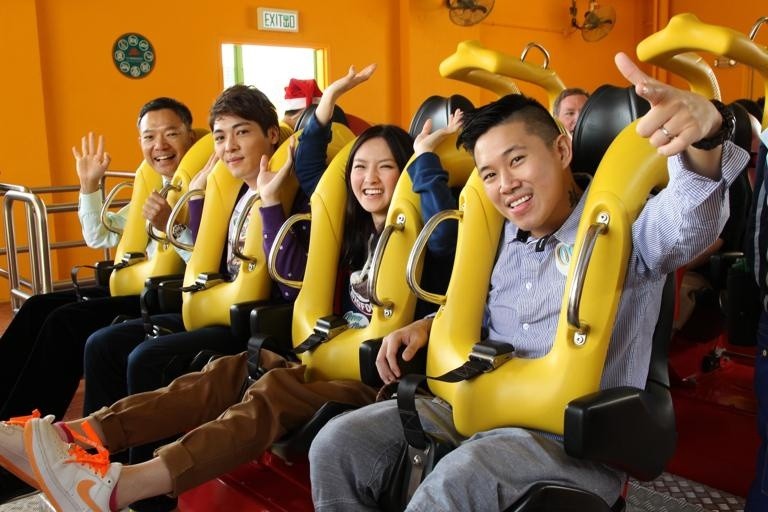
[660,125,674,140]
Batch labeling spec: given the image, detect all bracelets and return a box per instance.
[692,98,737,150]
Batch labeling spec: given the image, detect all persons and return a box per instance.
[550,81,768,512]
[307,53,748,511]
[1,62,467,512]
[281,77,349,134]
[81,84,308,510]
[0,97,198,500]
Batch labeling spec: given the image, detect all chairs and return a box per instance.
[392,82,682,512]
[92,114,217,341]
[244,91,475,511]
[154,102,355,433]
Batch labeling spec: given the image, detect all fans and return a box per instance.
[442,1,497,30]
[566,1,619,45]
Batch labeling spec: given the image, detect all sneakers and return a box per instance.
[0,408,122,512]
